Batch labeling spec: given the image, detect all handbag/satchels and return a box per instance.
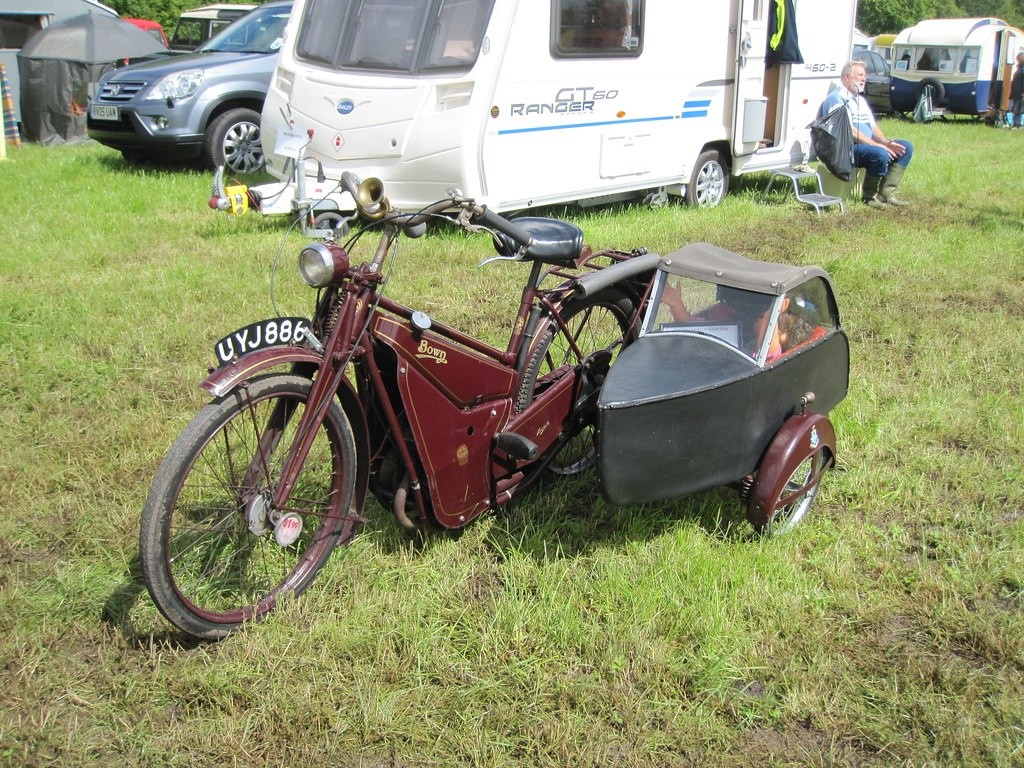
[1011,98,1024,114]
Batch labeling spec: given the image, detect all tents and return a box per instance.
[18,10,169,140]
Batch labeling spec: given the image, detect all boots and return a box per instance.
[861,170,887,208]
[877,162,910,206]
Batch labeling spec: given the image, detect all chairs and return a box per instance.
[813,162,867,204]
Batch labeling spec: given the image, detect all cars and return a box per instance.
[853,48,894,114]
[86,0,297,177]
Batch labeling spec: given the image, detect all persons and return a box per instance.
[822,61,913,208]
[660,282,798,362]
[1003,52,1024,130]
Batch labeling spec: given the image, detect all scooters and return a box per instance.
[138,170,664,641]
